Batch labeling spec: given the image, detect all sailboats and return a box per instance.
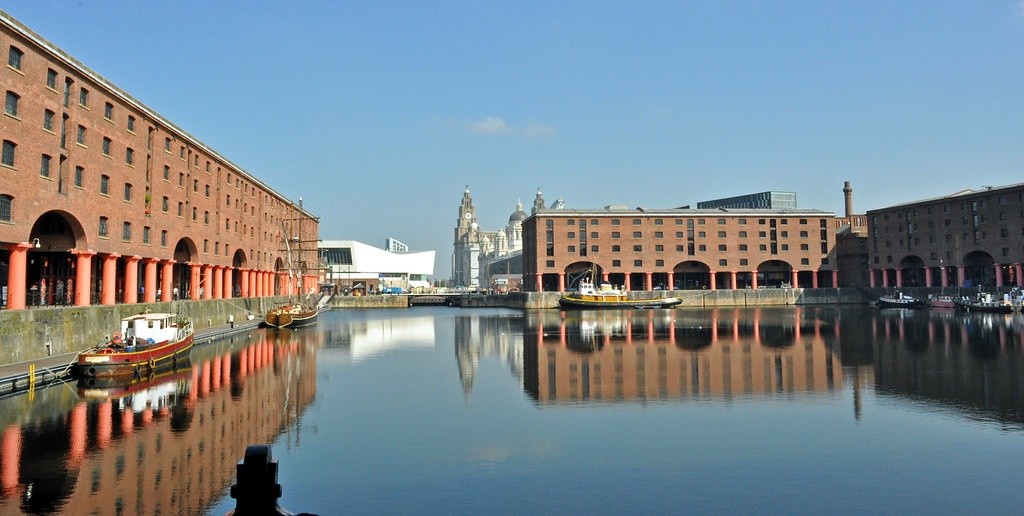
[264,198,333,330]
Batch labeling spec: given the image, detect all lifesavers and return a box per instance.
[173,353,178,361]
[88,366,97,376]
[104,348,114,354]
[136,374,142,382]
[136,365,141,373]
[148,372,155,382]
[149,357,155,369]
[87,378,96,387]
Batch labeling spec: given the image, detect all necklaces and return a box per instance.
[114,336,122,353]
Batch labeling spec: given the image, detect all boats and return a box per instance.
[79,305,196,376]
[955,284,1024,315]
[76,355,194,417]
[929,295,957,309]
[558,257,684,308]
[878,286,926,307]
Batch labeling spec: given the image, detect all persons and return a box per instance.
[158,288,161,299]
[139,284,144,302]
[173,286,178,300]
